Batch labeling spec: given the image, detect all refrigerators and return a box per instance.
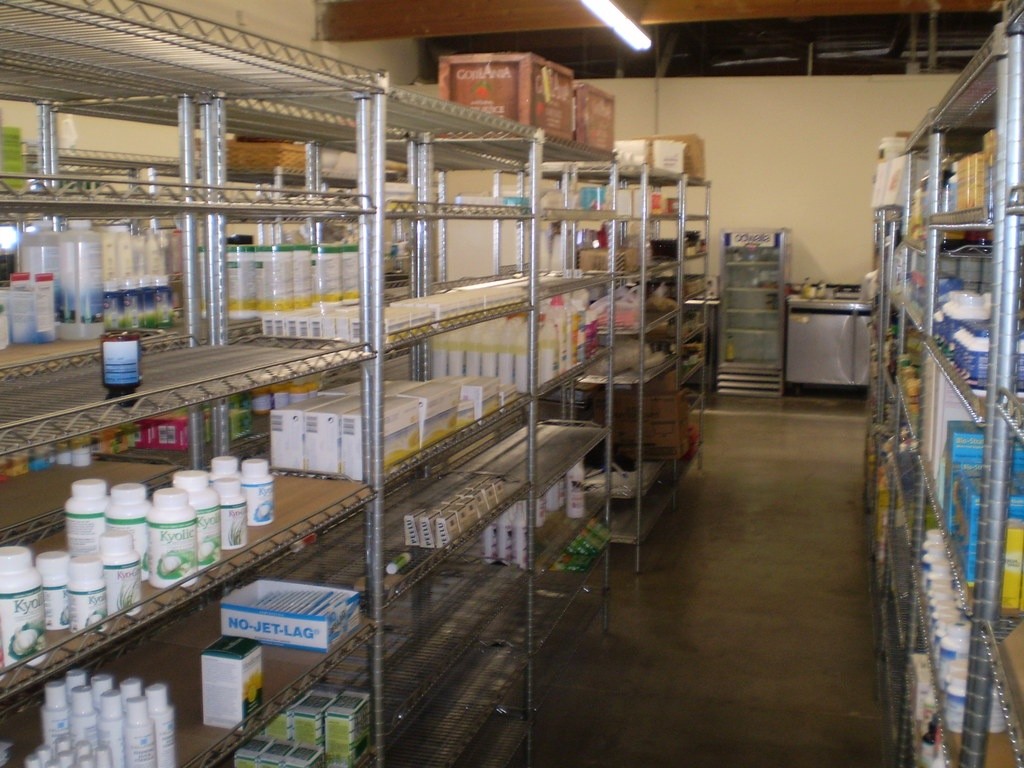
[715,226,785,398]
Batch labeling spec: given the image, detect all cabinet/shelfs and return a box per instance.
[717,227,793,396]
[0,0,713,768]
[863,0,1024,768]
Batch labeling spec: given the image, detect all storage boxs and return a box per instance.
[573,83,616,149]
[342,395,421,483]
[437,52,573,143]
[202,636,262,732]
[956,466,1024,618]
[943,417,1024,531]
[635,133,705,180]
[270,396,342,471]
[398,383,461,448]
[460,376,501,419]
[594,389,692,460]
[323,689,371,768]
[304,399,363,471]
[292,689,343,745]
[220,579,363,653]
[615,138,688,172]
[264,689,314,741]
[578,246,639,274]
[317,382,390,396]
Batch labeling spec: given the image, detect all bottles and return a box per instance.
[14,224,60,343]
[922,527,1009,733]
[22,670,176,768]
[932,279,1024,399]
[57,219,104,340]
[100,331,143,407]
[0,455,276,668]
[481,455,587,570]
[425,293,588,393]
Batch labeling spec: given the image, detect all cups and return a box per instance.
[194,242,374,321]
